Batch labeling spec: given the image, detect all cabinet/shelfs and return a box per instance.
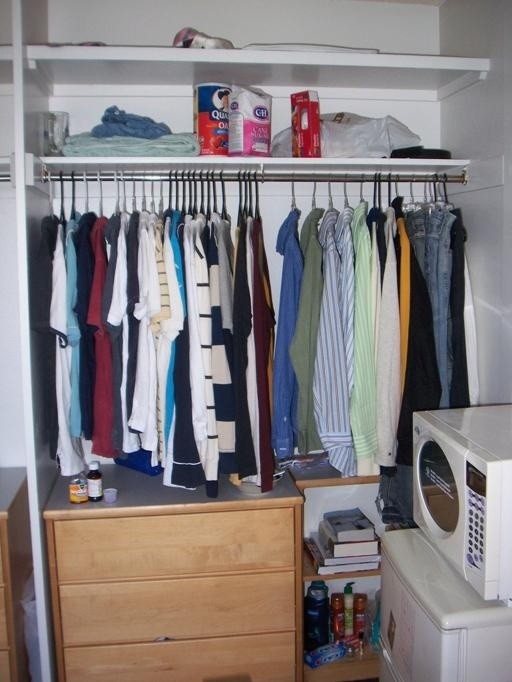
[24,42,490,184]
[0,466,36,680]
[44,461,305,681]
[290,460,389,682]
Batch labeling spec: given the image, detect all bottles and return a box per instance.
[330,593,344,639]
[86,460,103,502]
[353,594,366,637]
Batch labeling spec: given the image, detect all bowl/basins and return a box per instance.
[233,468,283,493]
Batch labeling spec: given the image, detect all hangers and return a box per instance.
[342,173,351,209]
[372,171,455,212]
[47,168,265,226]
[310,173,317,210]
[327,173,334,211]
[290,172,298,212]
[358,173,365,203]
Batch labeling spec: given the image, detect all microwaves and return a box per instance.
[412,403,512,602]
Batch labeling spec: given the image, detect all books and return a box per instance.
[302,507,382,575]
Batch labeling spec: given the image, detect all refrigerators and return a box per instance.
[377,528,512,682]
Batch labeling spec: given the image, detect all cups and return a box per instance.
[104,488,118,503]
[43,111,69,157]
